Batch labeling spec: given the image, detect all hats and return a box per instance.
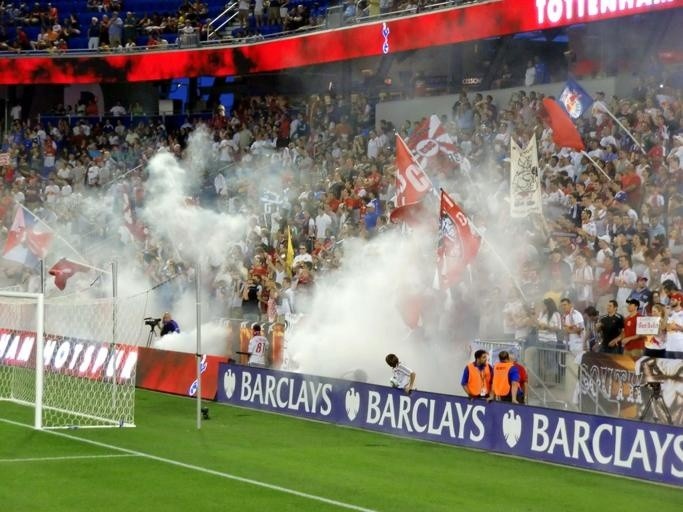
[626,299,640,306]
[636,276,648,282]
[253,325,261,331]
[615,190,628,203]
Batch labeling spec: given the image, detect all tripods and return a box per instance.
[144,326,157,349]
[639,390,673,425]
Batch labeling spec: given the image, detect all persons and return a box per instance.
[2,1,683,428]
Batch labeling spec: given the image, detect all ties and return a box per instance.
[570,207,574,216]
